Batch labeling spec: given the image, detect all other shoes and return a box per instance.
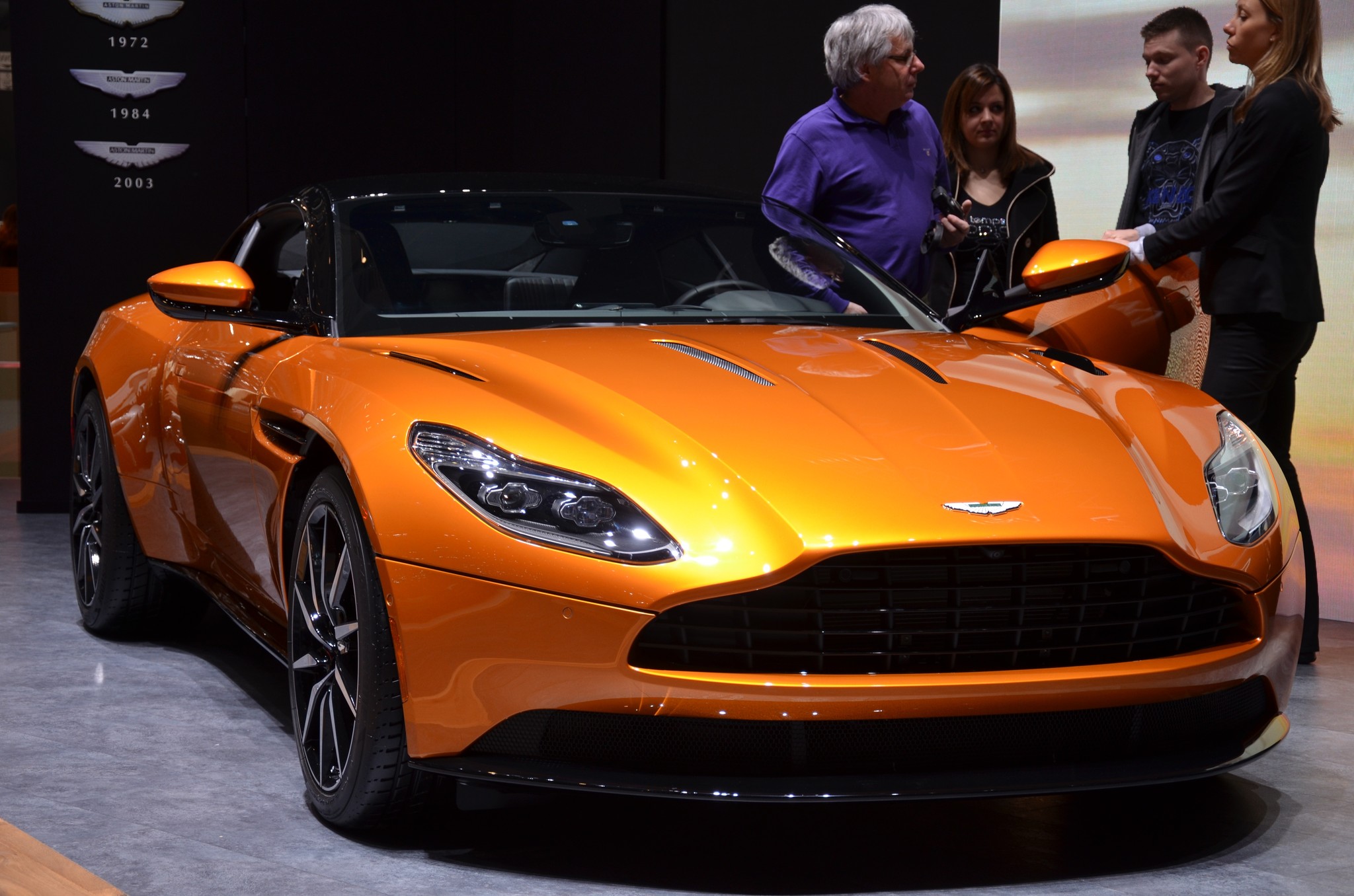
[1298,649,1316,665]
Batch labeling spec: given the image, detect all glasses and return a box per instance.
[883,50,916,67]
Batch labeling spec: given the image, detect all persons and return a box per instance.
[1101,0,1344,665]
[897,63,1060,333]
[762,3,972,314]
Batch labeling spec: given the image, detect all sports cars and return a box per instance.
[63,178,1309,841]
